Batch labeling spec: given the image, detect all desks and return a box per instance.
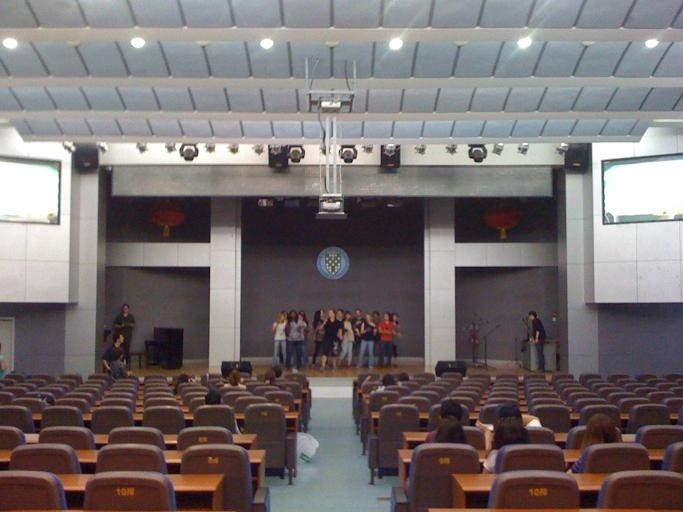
[522,341,558,372]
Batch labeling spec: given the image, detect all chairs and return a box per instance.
[144,340,158,366]
[0,365,319,512]
[346,367,682,512]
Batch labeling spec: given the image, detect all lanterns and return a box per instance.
[144,197,186,243]
[481,197,523,241]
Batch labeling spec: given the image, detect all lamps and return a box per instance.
[466,141,489,164]
[59,136,570,157]
[178,142,200,161]
[286,142,306,163]
[338,142,358,164]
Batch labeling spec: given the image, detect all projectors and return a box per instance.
[316,200,347,220]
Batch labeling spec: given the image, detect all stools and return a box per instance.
[125,351,148,368]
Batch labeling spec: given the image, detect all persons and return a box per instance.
[272,308,309,371]
[527,310,548,374]
[264,365,283,386]
[312,307,401,372]
[172,374,196,396]
[224,368,248,390]
[423,397,625,474]
[102,303,136,378]
[206,390,244,433]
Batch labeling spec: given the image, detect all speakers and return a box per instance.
[220,360,253,375]
[435,360,468,375]
[565,144,590,172]
[74,144,99,169]
